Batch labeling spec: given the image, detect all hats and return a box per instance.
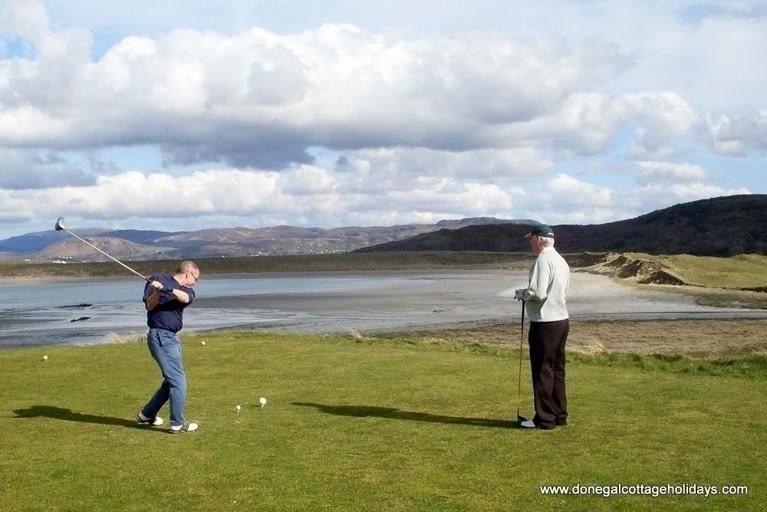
[524,224,555,240]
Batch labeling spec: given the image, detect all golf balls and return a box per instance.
[200,340,207,346]
[260,398,266,405]
[237,404,243,408]
[43,355,48,361]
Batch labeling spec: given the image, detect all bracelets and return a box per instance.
[159,283,174,295]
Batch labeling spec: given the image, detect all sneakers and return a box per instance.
[170,421,199,434]
[136,410,164,426]
[521,419,537,428]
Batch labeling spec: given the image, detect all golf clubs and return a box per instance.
[55,217,172,294]
[516,300,528,425]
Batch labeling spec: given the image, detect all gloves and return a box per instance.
[514,288,525,302]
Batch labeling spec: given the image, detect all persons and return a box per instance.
[514,224,571,428]
[135,260,200,433]
